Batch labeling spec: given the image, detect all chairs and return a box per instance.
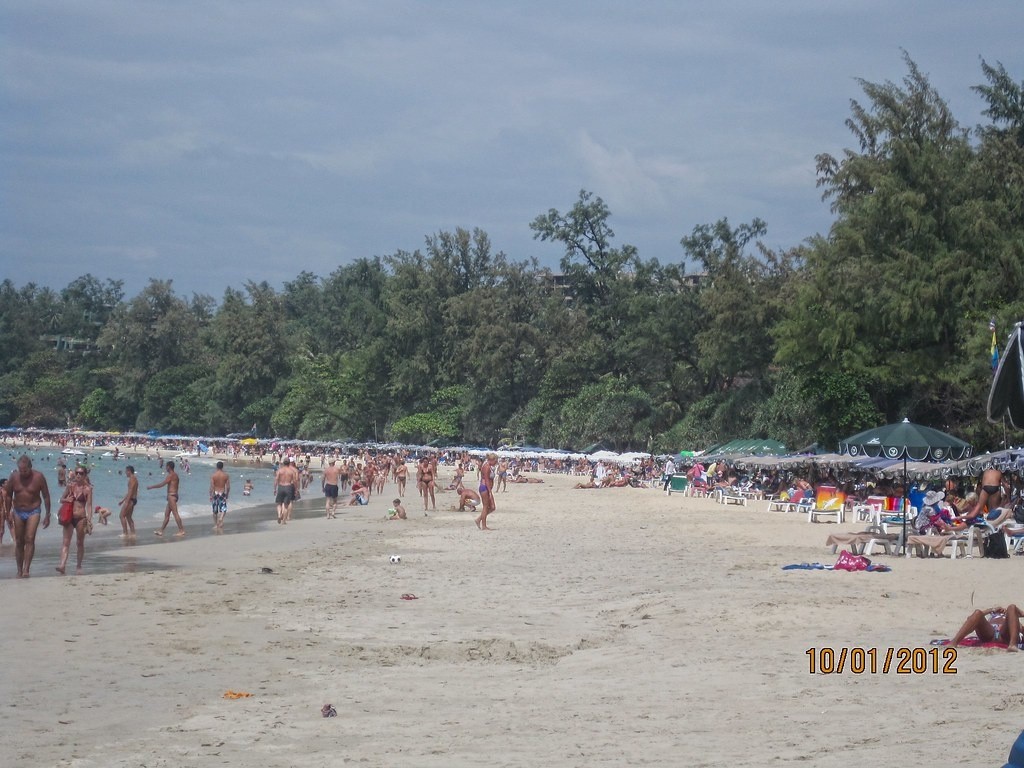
[522,467,1024,560]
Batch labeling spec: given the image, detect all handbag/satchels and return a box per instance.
[58,498,73,524]
[983,531,1011,559]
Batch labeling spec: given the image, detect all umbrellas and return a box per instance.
[835,417,974,552]
[1,426,1024,480]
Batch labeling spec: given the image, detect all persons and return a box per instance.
[949,604,1024,652]
[0,434,1024,579]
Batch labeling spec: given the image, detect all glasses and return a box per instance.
[74,472,85,476]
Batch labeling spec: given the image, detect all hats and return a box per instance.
[668,456,674,459]
[95,506,100,513]
[328,458,335,464]
[923,491,945,506]
